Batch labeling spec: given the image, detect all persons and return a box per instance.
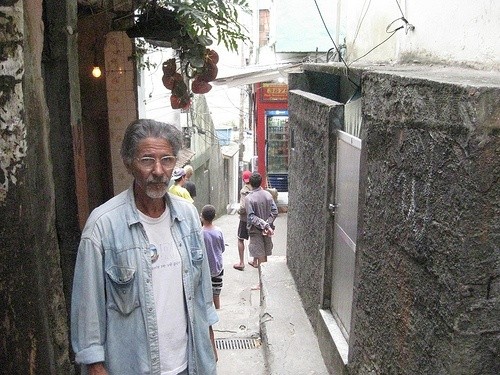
[244,174,279,264]
[70,119,219,375]
[200,204,225,309]
[168,168,195,203]
[232,170,258,270]
[181,164,196,199]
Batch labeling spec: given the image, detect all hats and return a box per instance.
[171,168,186,180]
[242,170,252,183]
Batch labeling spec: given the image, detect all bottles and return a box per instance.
[268,142,288,155]
[268,118,289,132]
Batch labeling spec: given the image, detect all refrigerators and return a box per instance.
[255,83,289,192]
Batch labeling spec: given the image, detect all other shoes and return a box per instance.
[233,263,245,270]
[248,261,258,268]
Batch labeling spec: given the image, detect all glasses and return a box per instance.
[133,155,178,170]
